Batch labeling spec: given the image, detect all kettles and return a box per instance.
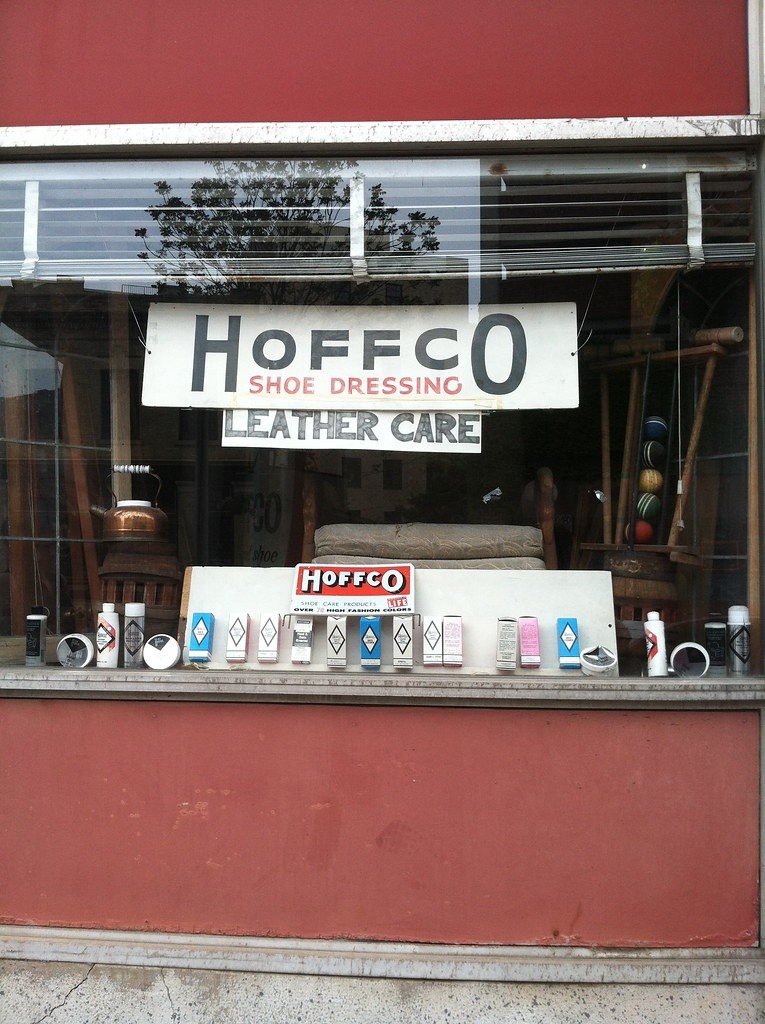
[88,463,169,537]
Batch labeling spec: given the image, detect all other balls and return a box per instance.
[624,414,668,544]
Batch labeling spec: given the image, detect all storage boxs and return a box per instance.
[392,616,414,670]
[257,612,282,664]
[225,611,251,664]
[326,616,348,669]
[557,617,581,670]
[360,616,382,670]
[290,615,315,665]
[188,612,215,663]
[443,614,463,667]
[422,616,443,667]
[495,616,518,671]
[518,615,541,669]
